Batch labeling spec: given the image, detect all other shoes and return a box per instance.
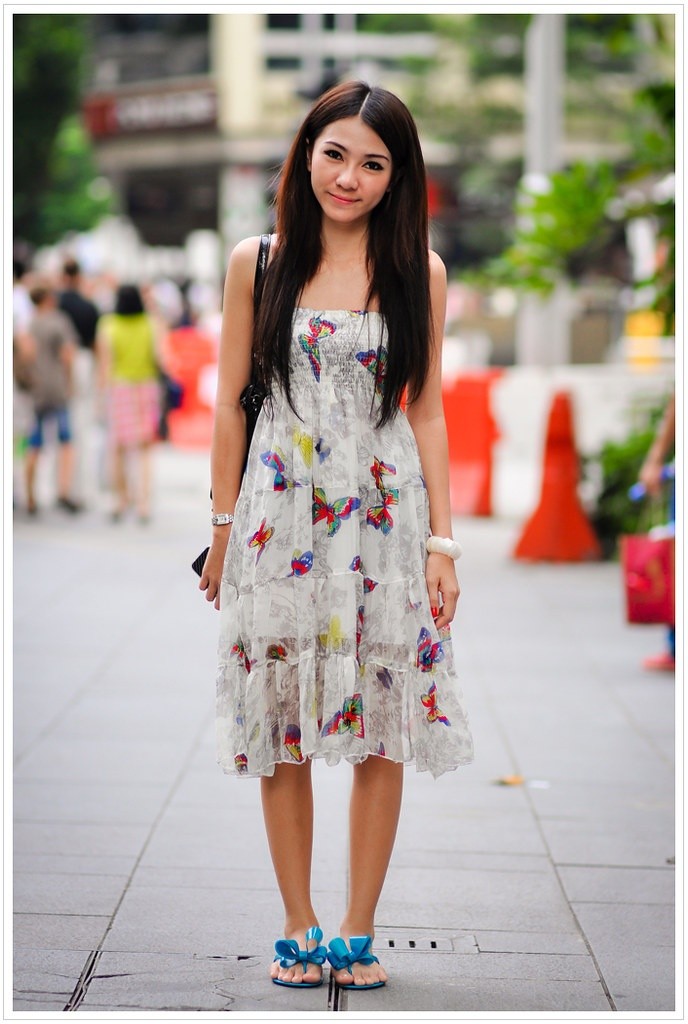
[642,654,672,673]
[26,507,40,517]
[53,495,82,516]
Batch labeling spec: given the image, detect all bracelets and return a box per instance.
[426,536,463,560]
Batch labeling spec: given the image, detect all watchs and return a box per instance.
[210,514,235,525]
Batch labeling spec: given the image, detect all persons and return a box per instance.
[637,394,676,669]
[202,82,464,994]
[14,248,220,525]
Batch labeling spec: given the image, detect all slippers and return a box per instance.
[326,933,385,990]
[271,925,324,988]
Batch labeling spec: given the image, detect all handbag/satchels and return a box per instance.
[617,488,676,624]
